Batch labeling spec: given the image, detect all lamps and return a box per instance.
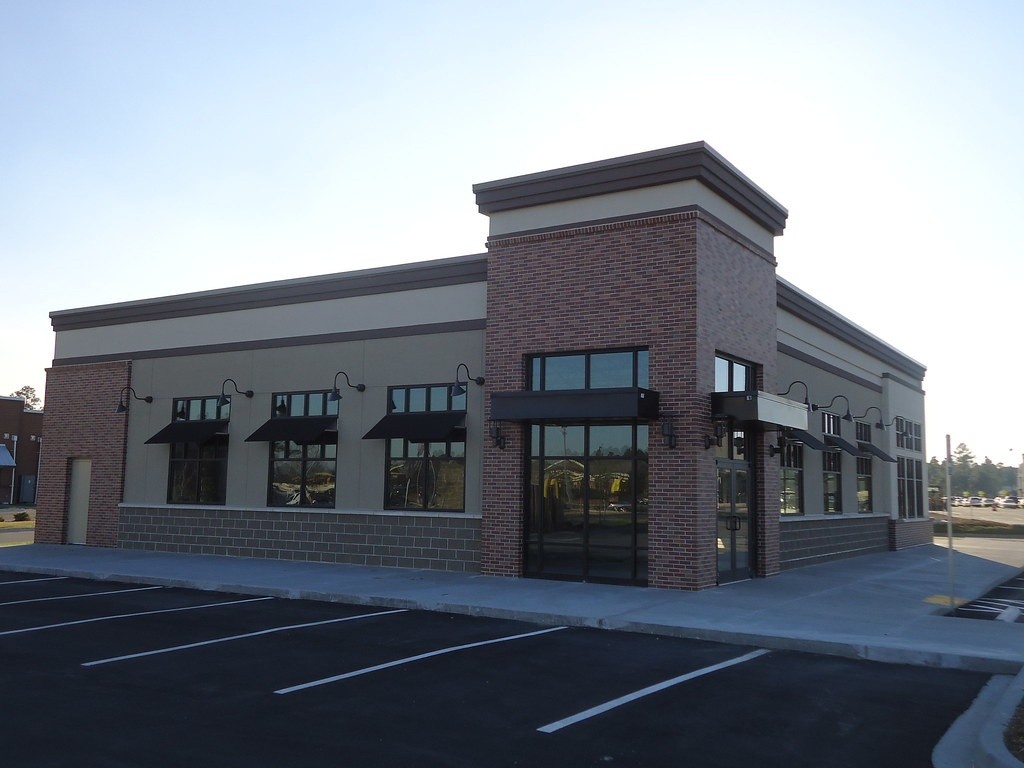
[812,395,855,423]
[216,379,254,406]
[328,372,365,401]
[116,386,153,413]
[885,415,911,438]
[662,421,676,450]
[705,421,726,449]
[770,437,785,456]
[776,381,813,413]
[853,407,886,431]
[451,363,484,396]
[487,418,505,449]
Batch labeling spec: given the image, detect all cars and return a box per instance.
[942,495,1024,509]
[788,496,796,510]
[390,463,406,484]
[828,495,833,508]
[310,473,335,503]
